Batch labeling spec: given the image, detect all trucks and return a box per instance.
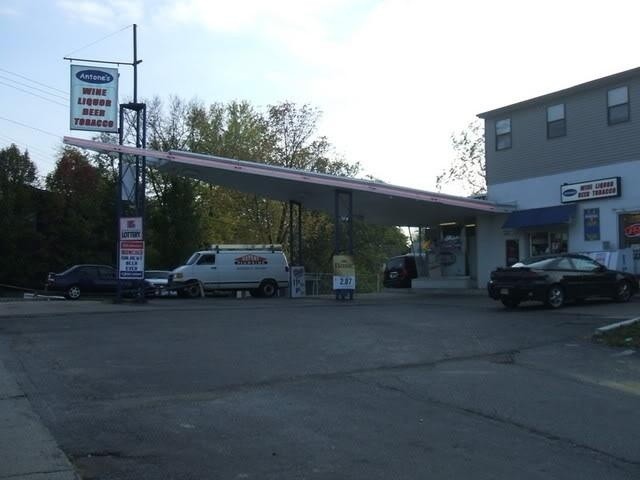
[163,241,293,298]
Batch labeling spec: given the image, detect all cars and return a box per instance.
[47,264,154,304]
[143,268,177,295]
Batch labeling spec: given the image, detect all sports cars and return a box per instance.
[486,251,638,311]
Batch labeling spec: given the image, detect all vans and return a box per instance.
[382,253,427,291]
